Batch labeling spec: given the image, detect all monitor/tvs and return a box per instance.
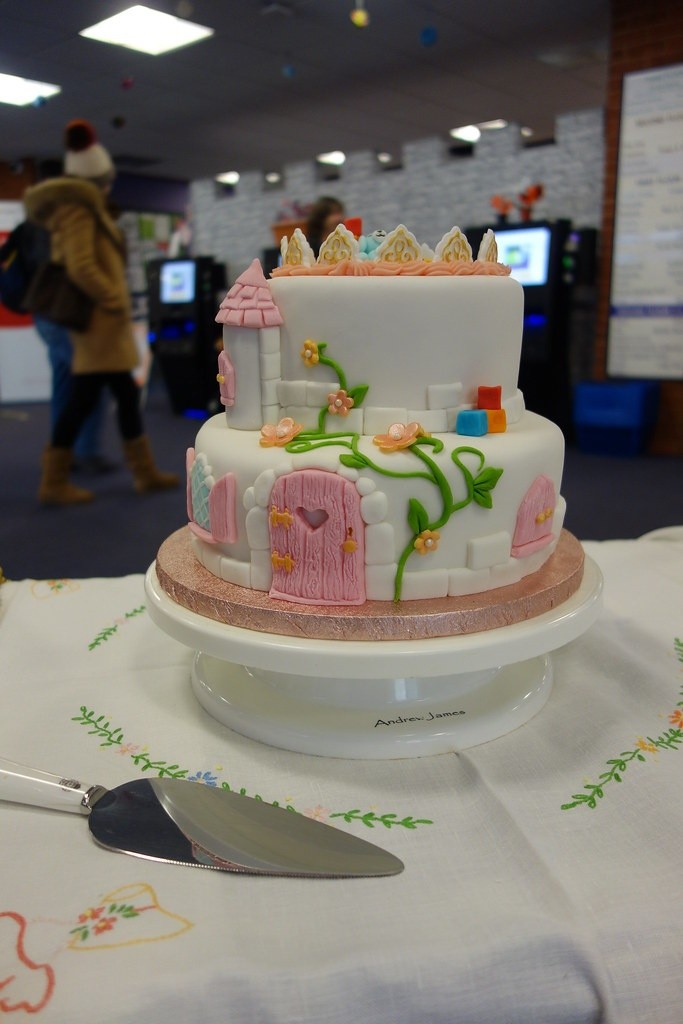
[158,258,198,306]
[491,219,556,286]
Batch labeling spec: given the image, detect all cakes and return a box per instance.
[152,224,586,640]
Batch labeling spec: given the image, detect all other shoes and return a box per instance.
[69,453,120,476]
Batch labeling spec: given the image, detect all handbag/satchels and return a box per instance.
[26,256,96,333]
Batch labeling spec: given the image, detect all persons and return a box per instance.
[21,121,187,508]
[28,154,124,483]
[303,195,347,263]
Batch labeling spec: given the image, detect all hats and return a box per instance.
[63,119,116,188]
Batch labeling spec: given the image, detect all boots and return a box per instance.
[123,434,181,494]
[39,446,95,506]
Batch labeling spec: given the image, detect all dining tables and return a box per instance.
[0,526,683,1024]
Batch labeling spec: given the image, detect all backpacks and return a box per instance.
[0,220,50,313]
